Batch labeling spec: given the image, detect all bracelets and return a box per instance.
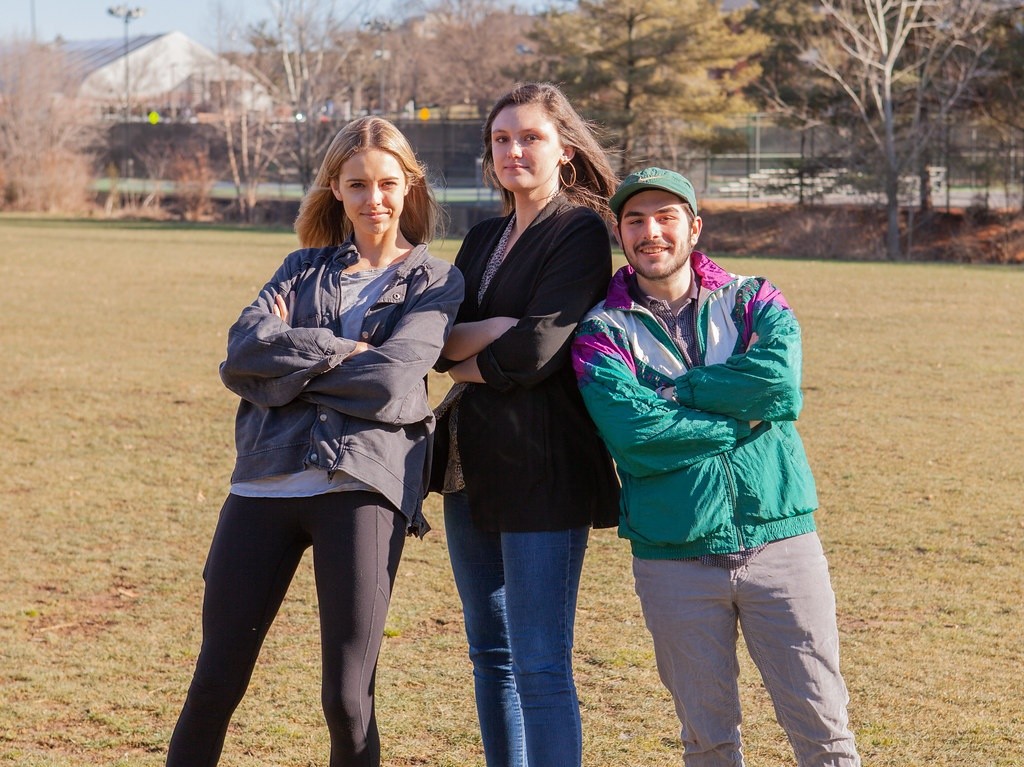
[653,385,666,401]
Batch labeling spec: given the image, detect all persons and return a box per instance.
[568,166,867,767]
[164,113,468,767]
[422,82,627,767]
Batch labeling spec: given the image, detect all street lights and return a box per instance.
[113,8,143,175]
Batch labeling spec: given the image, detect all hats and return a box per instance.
[609,167,697,218]
